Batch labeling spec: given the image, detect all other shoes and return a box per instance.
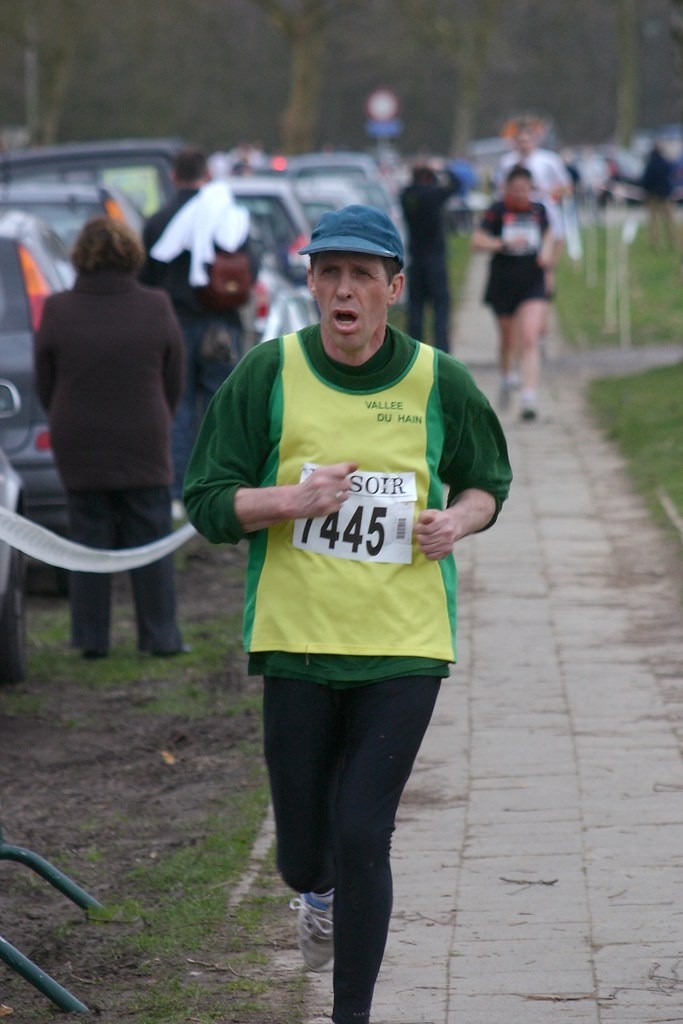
[81,647,110,661]
[149,642,191,661]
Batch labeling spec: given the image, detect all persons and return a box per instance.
[492,124,571,366]
[232,143,253,177]
[31,218,193,659]
[138,148,259,521]
[399,167,461,355]
[641,144,677,258]
[471,167,552,424]
[561,144,618,206]
[180,205,513,1024]
[425,153,477,231]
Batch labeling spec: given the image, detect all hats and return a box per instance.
[173,145,209,181]
[299,205,405,270]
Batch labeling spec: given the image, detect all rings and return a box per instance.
[336,491,343,501]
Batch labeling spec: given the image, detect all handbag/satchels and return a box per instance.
[212,249,252,311]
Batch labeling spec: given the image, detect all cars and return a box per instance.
[0,136,649,687]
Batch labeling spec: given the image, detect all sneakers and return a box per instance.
[289,891,335,971]
